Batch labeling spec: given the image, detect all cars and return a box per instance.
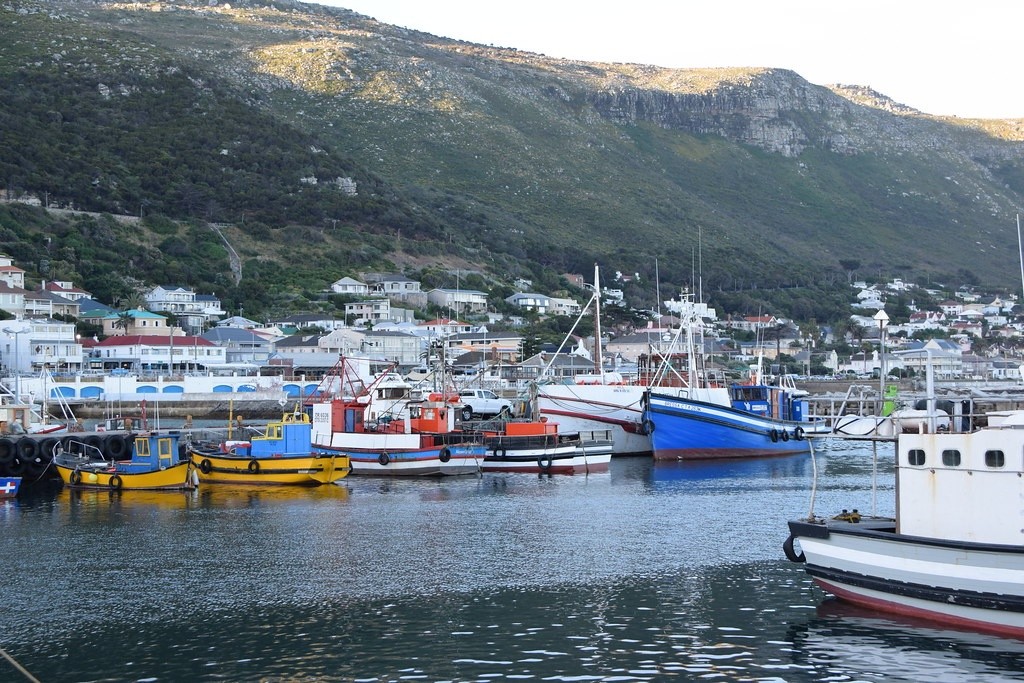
[453,365,479,376]
[457,388,515,421]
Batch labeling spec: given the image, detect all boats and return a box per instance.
[0,477,23,499]
[95,375,182,432]
[299,328,829,477]
[52,388,196,491]
[0,364,79,436]
[178,396,352,487]
[782,214,1024,642]
